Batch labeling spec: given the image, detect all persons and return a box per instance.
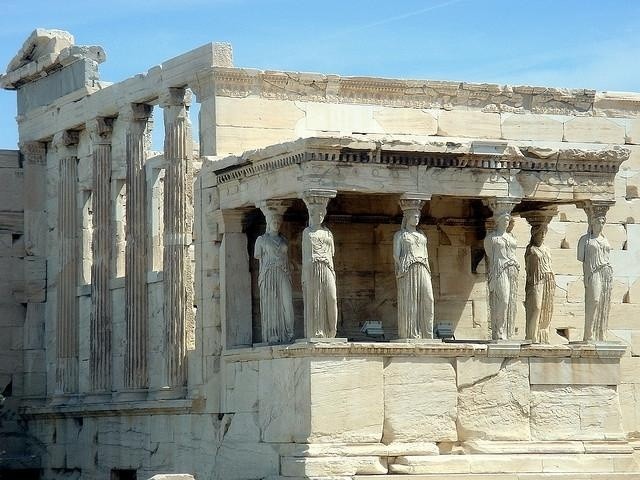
[483,203,521,340]
[524,217,556,342]
[392,202,435,339]
[577,208,614,339]
[255,207,296,345]
[301,199,338,339]
